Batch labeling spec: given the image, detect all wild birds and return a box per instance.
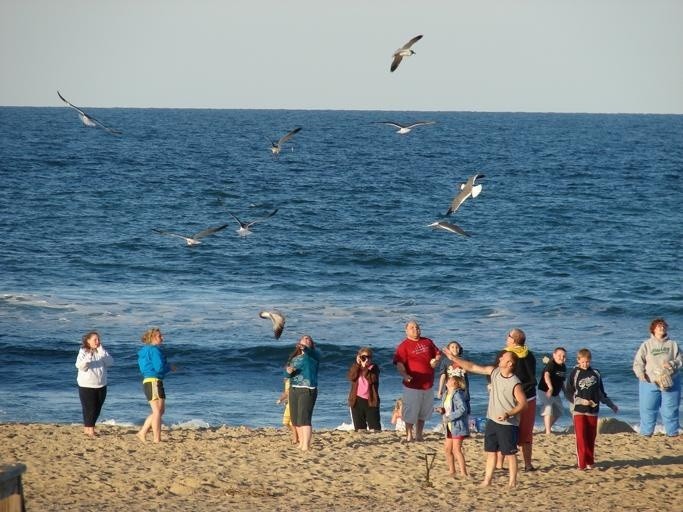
[56,91,121,135]
[389,34,423,73]
[258,310,284,340]
[216,197,278,239]
[151,224,228,245]
[427,221,471,239]
[369,120,437,135]
[445,173,485,217]
[247,126,302,154]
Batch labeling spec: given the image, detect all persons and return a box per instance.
[442,347,527,489]
[75,332,113,439]
[348,347,381,431]
[135,328,176,446]
[565,349,618,470]
[436,375,470,476]
[392,399,406,431]
[487,328,537,473]
[393,321,441,441]
[632,318,683,437]
[280,335,321,451]
[538,347,567,434]
[439,341,470,414]
